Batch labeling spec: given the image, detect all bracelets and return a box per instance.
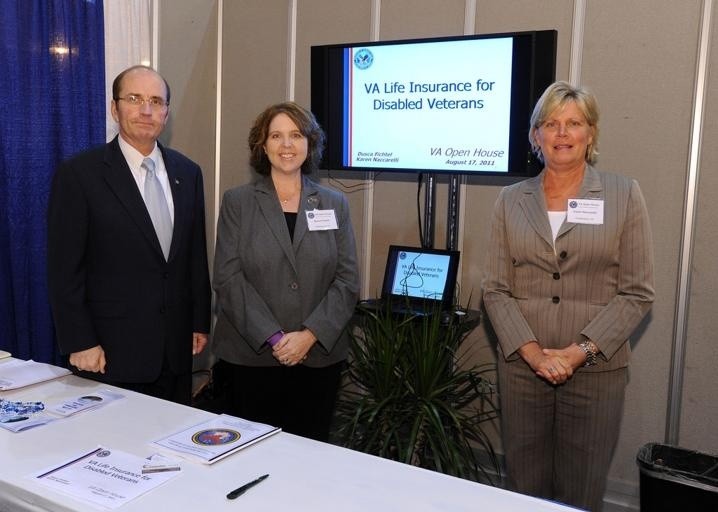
[580,340,597,367]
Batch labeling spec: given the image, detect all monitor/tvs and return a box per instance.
[310,29,559,177]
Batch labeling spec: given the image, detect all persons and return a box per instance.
[48,66,214,403]
[479,81,656,511]
[212,100,361,441]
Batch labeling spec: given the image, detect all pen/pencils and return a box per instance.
[141,466,180,475]
[226,473,270,500]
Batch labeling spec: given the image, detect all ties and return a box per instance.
[141,157,173,263]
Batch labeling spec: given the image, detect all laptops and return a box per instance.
[359,243,461,322]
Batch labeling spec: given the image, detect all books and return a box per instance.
[0,348,72,392]
[151,413,283,464]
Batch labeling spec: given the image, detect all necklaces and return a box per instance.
[268,174,300,204]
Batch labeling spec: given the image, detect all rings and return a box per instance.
[548,366,556,374]
[77,368,83,371]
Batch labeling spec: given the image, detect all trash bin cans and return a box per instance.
[636,441,718,512]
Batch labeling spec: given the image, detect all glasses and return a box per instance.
[120,95,169,109]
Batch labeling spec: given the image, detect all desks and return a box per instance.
[354,309,482,334]
[0,358,590,512]
